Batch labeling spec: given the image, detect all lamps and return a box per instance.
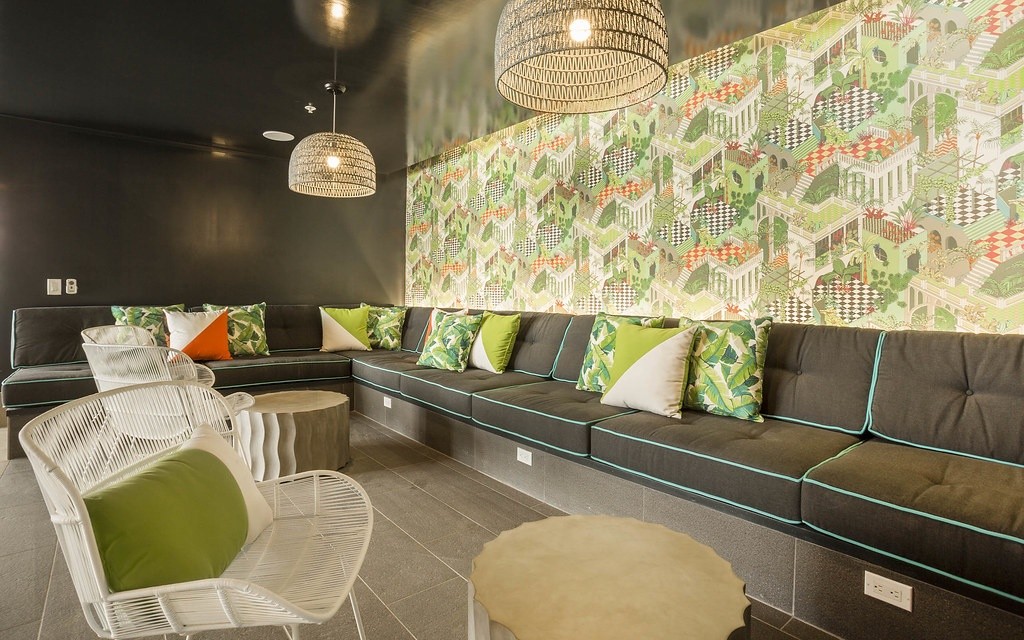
[289,82,376,198]
[493,0,669,113]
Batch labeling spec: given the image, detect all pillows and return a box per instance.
[416,307,522,374]
[576,312,773,423]
[81,420,275,583]
[319,301,409,352]
[110,302,271,360]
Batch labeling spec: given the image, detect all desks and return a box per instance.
[467,515,753,640]
[237,390,350,481]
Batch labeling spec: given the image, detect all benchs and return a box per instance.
[352,302,1024,640]
[1,302,354,460]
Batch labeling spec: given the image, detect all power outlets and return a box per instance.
[66,279,77,294]
[864,571,913,613]
[517,447,532,466]
[384,397,391,408]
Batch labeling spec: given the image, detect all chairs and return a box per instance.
[81,343,255,490]
[81,325,215,457]
[18,379,375,640]
